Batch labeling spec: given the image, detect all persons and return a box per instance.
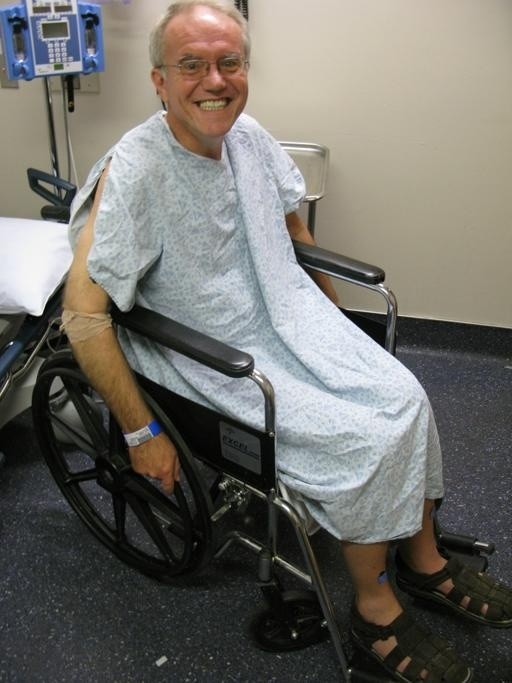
[58,0,512,683]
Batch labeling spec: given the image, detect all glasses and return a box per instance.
[154,58,250,80]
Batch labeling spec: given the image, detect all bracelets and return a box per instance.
[123,418,164,450]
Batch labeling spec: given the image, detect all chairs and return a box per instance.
[33,143,494,628]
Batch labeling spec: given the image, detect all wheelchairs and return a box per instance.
[28,239,496,683]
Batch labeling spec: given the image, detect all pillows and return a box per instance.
[0,212,84,321]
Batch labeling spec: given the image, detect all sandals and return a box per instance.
[351,595,474,682]
[395,543,512,629]
